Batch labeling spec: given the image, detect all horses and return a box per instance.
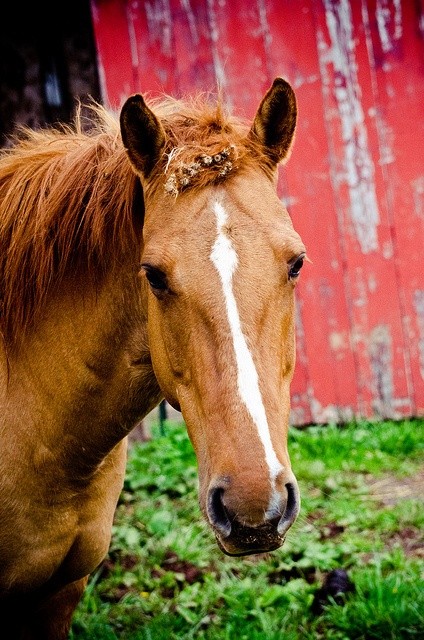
[0,75,315,640]
